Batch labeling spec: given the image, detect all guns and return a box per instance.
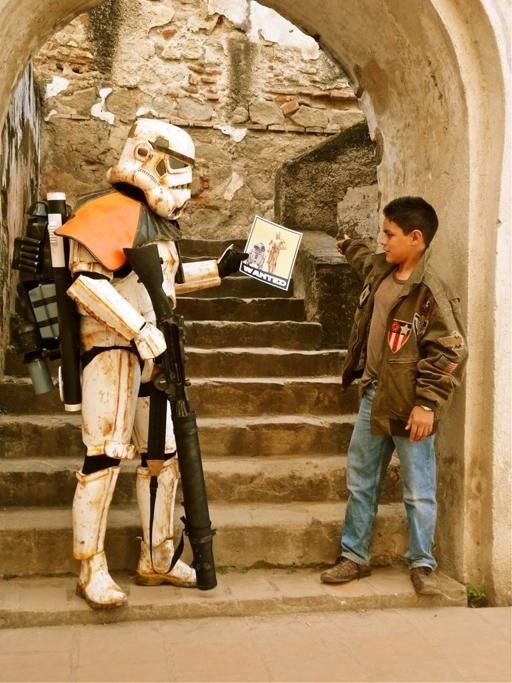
[122,244,217,589]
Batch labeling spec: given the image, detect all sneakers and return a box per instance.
[134,536,199,590]
[320,556,373,585]
[74,551,129,611]
[409,565,441,596]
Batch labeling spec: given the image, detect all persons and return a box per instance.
[52,118,251,611]
[319,195,469,596]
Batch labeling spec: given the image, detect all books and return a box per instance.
[238,214,305,293]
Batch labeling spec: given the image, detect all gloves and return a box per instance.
[218,249,250,278]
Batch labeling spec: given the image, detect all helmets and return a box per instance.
[104,115,197,222]
